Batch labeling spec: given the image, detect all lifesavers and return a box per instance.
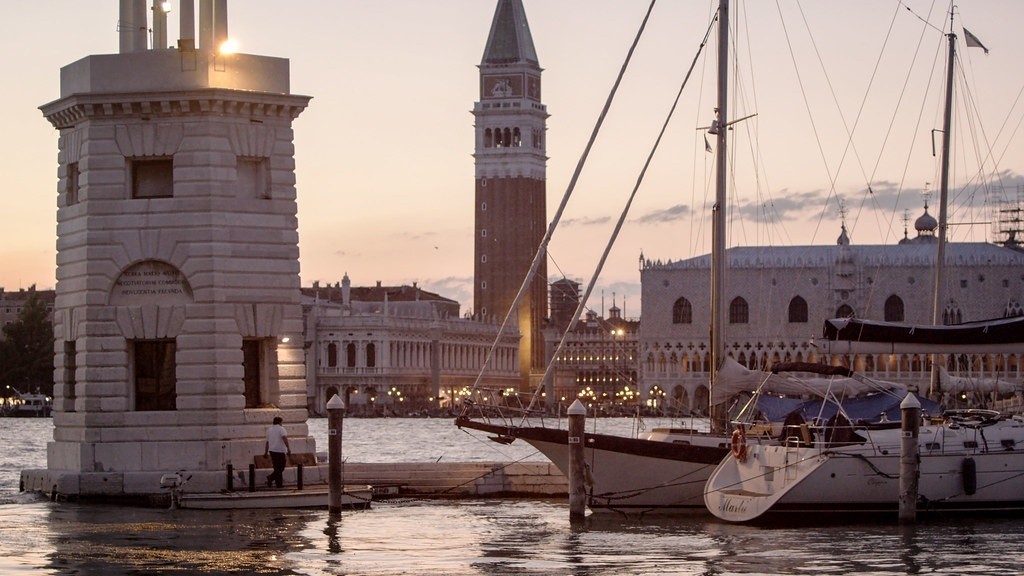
[731,430,746,458]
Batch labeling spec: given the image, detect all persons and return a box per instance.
[260,414,297,491]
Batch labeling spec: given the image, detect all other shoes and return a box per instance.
[276,484,285,488]
[267,475,272,488]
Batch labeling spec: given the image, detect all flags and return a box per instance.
[703,135,712,153]
[963,27,988,54]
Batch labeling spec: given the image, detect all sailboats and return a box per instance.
[452,0,1024,525]
